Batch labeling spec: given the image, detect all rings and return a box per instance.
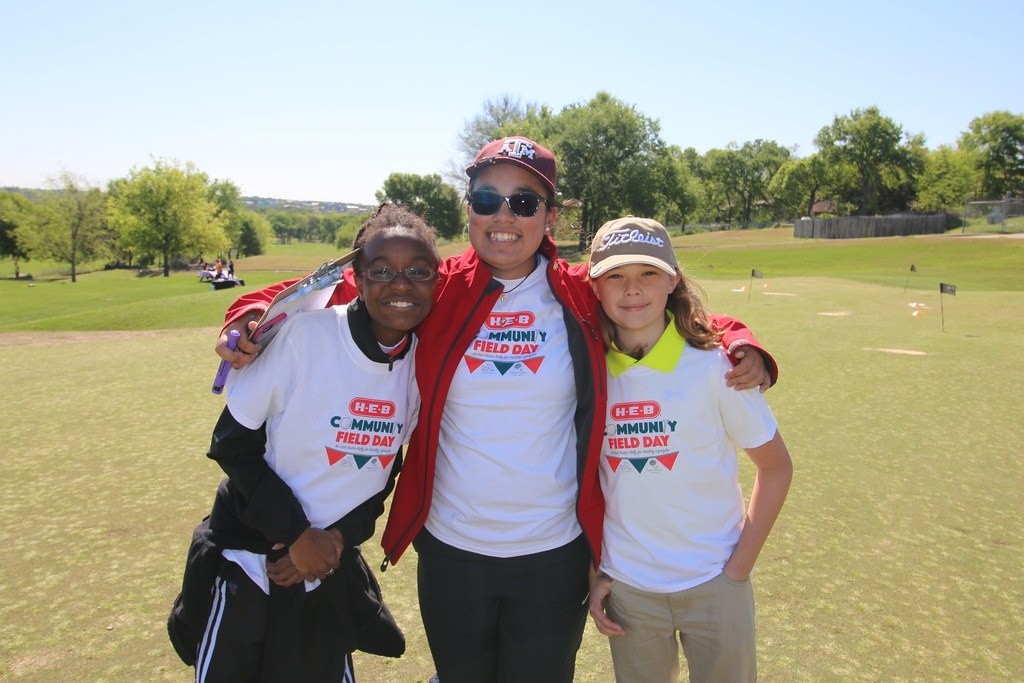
[324,569,333,574]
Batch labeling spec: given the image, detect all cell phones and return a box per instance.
[251,313,288,344]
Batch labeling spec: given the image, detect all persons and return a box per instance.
[214,136,778,683]
[586,215,793,683]
[195,202,440,683]
[15,263,19,278]
[198,257,234,281]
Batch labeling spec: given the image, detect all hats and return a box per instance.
[465,135,557,194]
[590,215,679,279]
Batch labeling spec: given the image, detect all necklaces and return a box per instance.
[496,266,538,328]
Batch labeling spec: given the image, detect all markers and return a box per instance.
[211,329,240,395]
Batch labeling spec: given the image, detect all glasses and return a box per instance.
[466,188,552,218]
[358,265,438,282]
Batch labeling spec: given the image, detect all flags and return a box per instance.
[752,269,762,279]
[940,283,956,295]
[911,265,917,272]
